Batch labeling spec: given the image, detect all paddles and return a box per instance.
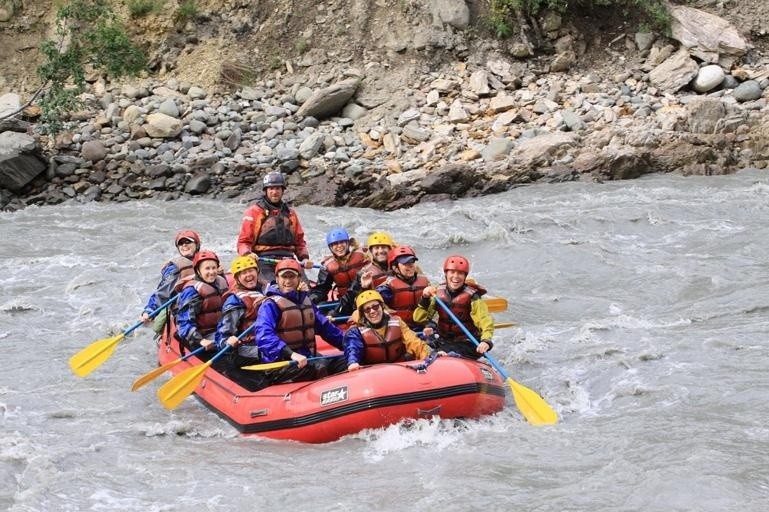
[416,323,520,335]
[429,289,558,427]
[483,299,508,313]
[241,353,346,372]
[68,293,183,376]
[131,342,206,393]
[159,321,256,410]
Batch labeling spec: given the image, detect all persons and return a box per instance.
[214,256,272,367]
[309,227,371,306]
[255,258,348,385]
[326,232,396,319]
[142,230,226,325]
[176,252,235,352]
[342,290,448,371]
[361,246,432,329]
[413,255,494,361]
[237,171,314,285]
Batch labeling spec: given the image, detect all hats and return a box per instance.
[396,255,418,264]
[277,269,298,276]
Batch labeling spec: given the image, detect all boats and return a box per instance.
[158,269,504,444]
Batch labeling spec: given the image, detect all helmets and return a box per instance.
[231,257,259,281]
[356,290,384,311]
[262,172,286,191]
[444,256,469,274]
[274,259,303,277]
[175,230,201,246]
[192,251,219,274]
[326,228,349,246]
[367,233,393,251]
[387,246,416,269]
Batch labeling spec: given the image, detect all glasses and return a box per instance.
[364,305,380,313]
[177,239,192,245]
